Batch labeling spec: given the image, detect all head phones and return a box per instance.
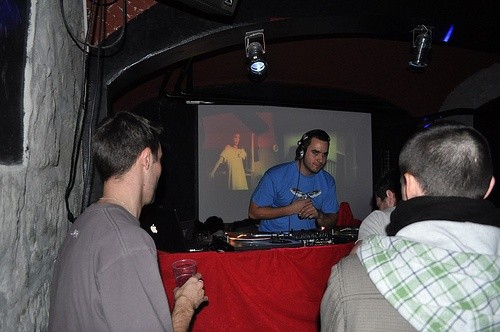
[295,131,330,159]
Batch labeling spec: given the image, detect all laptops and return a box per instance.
[139,207,214,252]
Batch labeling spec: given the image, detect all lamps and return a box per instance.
[402,21,437,73]
[245,27,269,78]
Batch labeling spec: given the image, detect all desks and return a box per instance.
[155,242,359,332]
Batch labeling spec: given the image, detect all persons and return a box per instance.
[248,129,339,232]
[210,130,248,190]
[317,125,500,332]
[48,111,209,332]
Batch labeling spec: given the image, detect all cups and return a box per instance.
[171,260,197,288]
